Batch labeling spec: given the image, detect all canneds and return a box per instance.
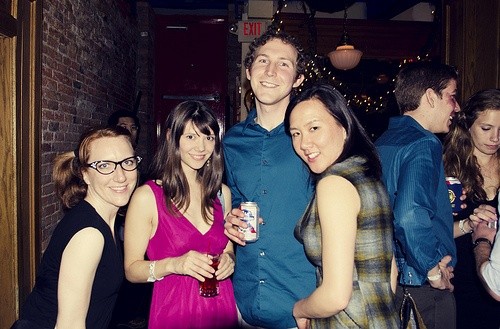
[237,201,259,242]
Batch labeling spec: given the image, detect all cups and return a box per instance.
[199,251,220,297]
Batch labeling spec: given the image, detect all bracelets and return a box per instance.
[459,218,473,234]
[147,260,164,283]
[472,237,493,250]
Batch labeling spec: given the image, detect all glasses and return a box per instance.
[84,155,143,175]
[121,124,139,133]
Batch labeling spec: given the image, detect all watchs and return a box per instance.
[427,263,443,281]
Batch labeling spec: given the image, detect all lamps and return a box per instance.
[328,9,363,70]
[229,19,272,43]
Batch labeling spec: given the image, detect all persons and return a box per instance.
[9,124,142,329]
[106,111,146,329]
[125,100,240,329]
[375,60,497,329]
[283,81,402,329]
[220,32,316,329]
[471,187,500,302]
[443,89,500,329]
